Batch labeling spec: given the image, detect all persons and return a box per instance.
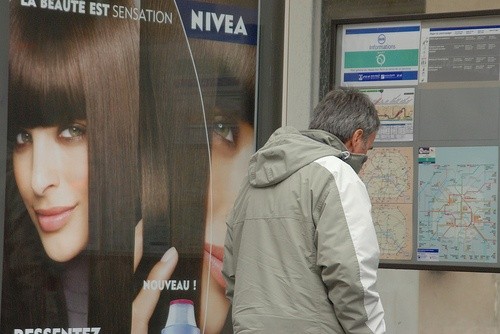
[133,0,260,334]
[220,84,387,334]
[0,0,133,334]
